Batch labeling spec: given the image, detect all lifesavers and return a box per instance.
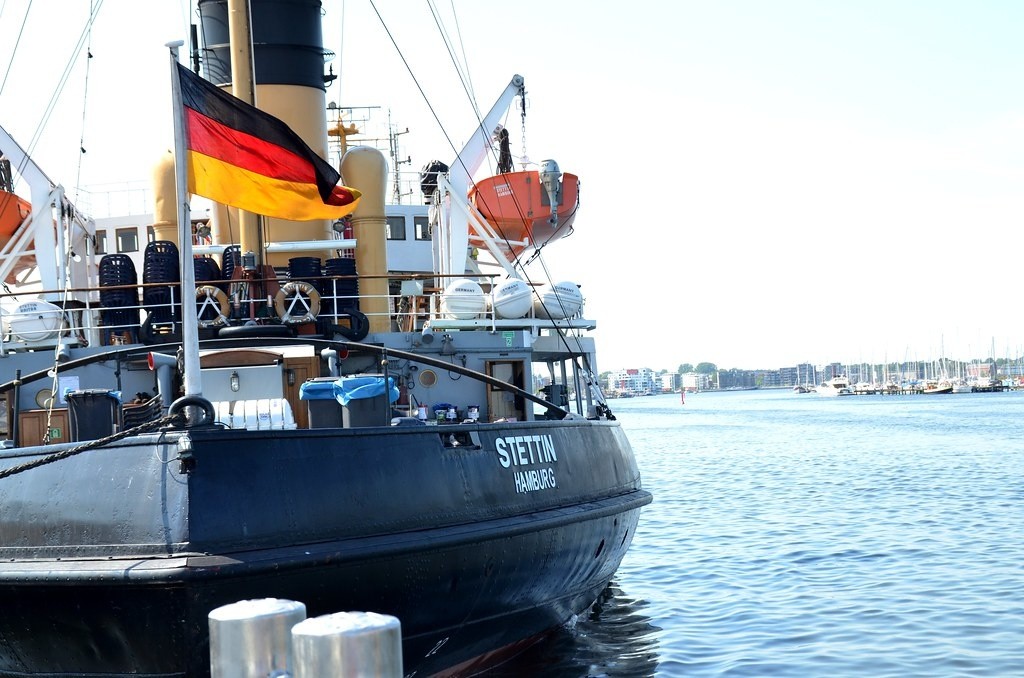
[193,285,232,331]
[274,281,321,327]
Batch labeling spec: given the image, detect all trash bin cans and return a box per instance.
[65,387,116,442]
[299,376,342,428]
[336,374,394,427]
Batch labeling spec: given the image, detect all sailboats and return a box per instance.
[681,392,685,404]
[971,336,1013,392]
[792,364,806,394]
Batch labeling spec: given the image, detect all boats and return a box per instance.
[903,385,922,394]
[642,392,656,396]
[837,388,856,396]
[603,394,618,399]
[923,381,954,395]
[661,388,676,394]
[854,383,875,395]
[816,374,849,391]
[618,391,635,398]
[0,0,653,678]
[881,380,902,395]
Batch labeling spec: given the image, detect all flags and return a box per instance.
[176,61,362,222]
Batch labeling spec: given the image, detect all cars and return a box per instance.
[960,376,978,386]
